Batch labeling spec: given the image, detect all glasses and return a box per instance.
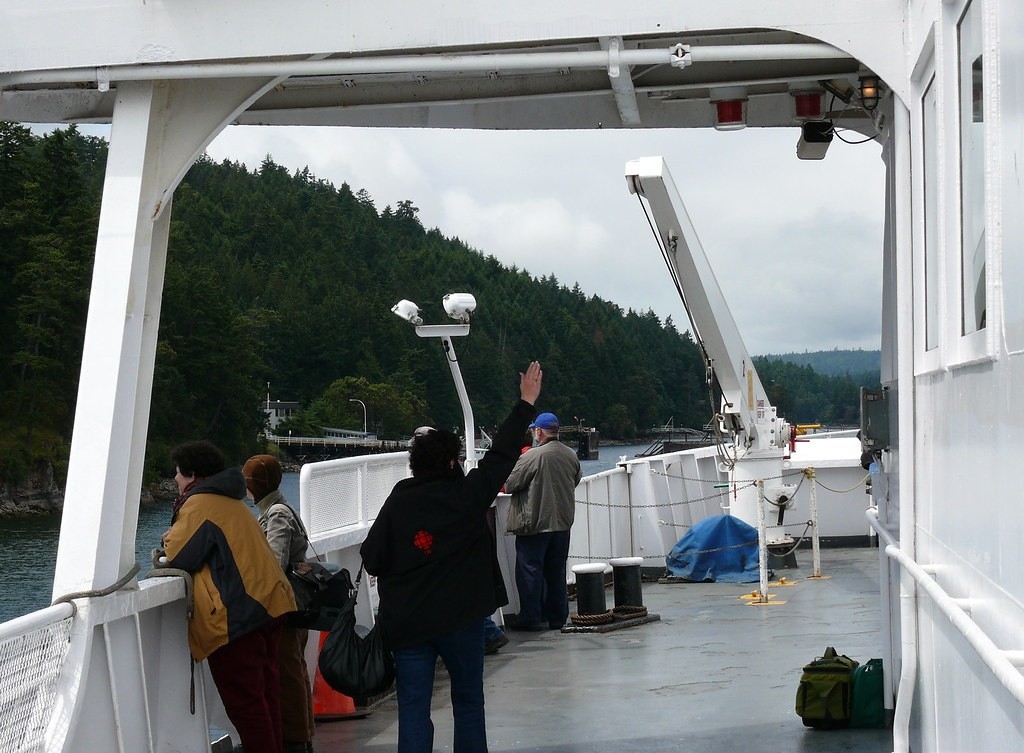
[530,427,538,431]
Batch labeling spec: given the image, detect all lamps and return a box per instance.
[857,76,882,110]
[390,300,424,325]
[442,292,476,323]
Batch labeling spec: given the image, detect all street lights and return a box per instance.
[350,399,366,439]
[580,419,585,430]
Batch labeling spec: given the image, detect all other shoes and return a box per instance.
[548,621,564,629]
[484,633,510,655]
[510,619,549,630]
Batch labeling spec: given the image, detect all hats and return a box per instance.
[405,426,438,448]
[242,455,282,505]
[529,412,560,430]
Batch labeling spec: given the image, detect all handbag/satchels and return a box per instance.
[262,503,353,631]
[852,658,886,728]
[318,561,395,699]
[795,647,860,729]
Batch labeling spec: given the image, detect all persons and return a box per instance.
[241,454,316,753]
[499,411,583,632]
[161,438,299,753]
[359,359,543,753]
